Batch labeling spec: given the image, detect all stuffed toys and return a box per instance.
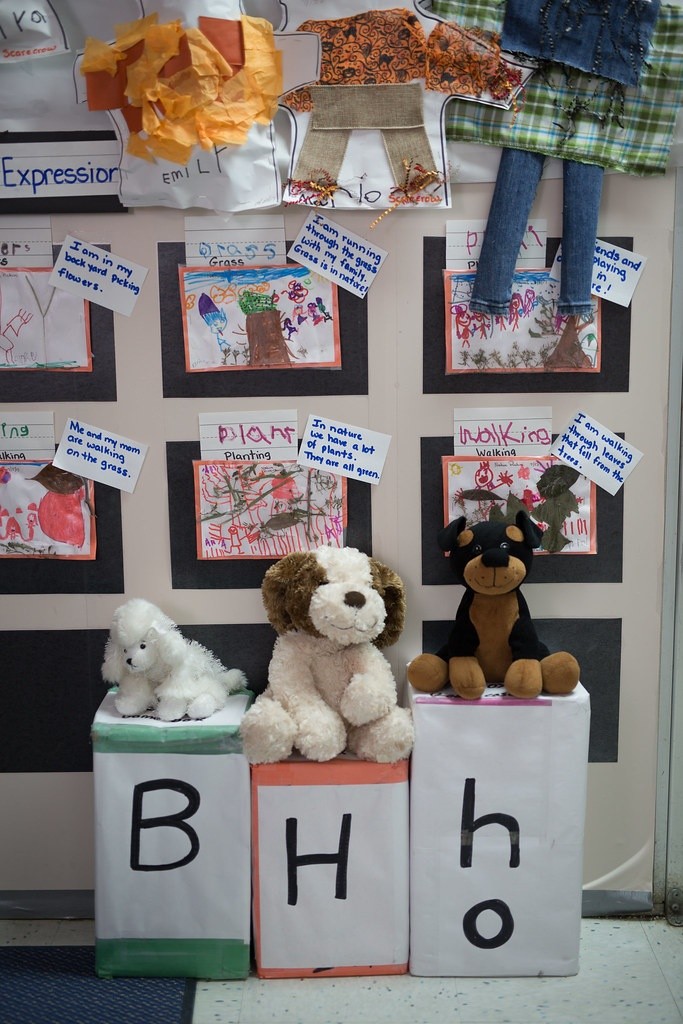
[406,509,581,700]
[102,598,248,722]
[239,545,415,766]
